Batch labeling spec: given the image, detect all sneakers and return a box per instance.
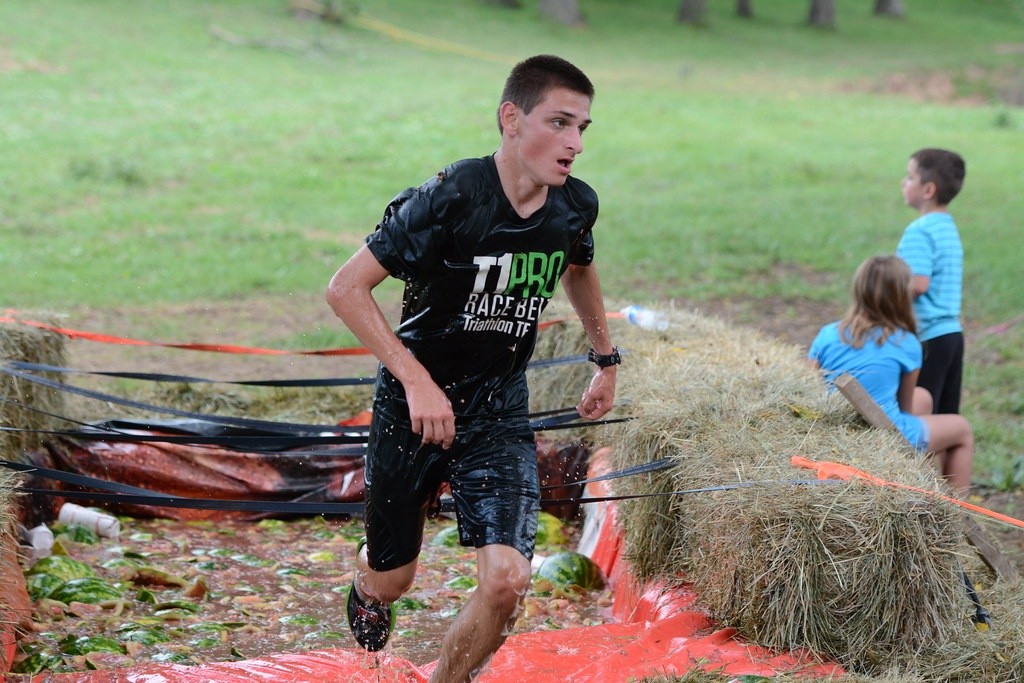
[345,536,397,654]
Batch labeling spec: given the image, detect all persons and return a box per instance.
[808,256,973,499]
[326,54,622,683]
[896,148,965,413]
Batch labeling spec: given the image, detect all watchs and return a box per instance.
[588,346,622,367]
